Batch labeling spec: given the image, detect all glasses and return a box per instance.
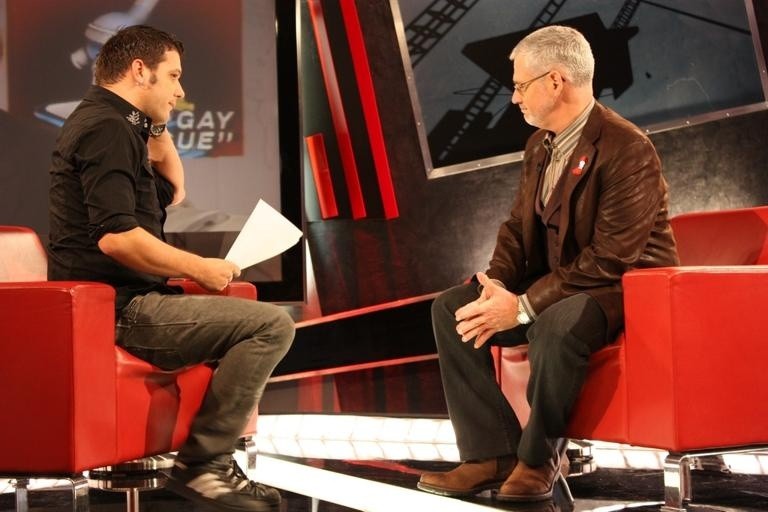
[513,71,566,95]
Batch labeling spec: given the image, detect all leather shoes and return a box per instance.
[417,447,561,502]
[516,500,561,512]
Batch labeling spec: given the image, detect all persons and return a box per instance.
[416,25,679,504]
[47,25,297,511]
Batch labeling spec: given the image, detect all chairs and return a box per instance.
[464,207,767,488]
[1,221,258,489]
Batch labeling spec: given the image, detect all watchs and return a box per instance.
[149,122,165,137]
[516,297,531,324]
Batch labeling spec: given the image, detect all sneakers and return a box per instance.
[165,453,281,511]
